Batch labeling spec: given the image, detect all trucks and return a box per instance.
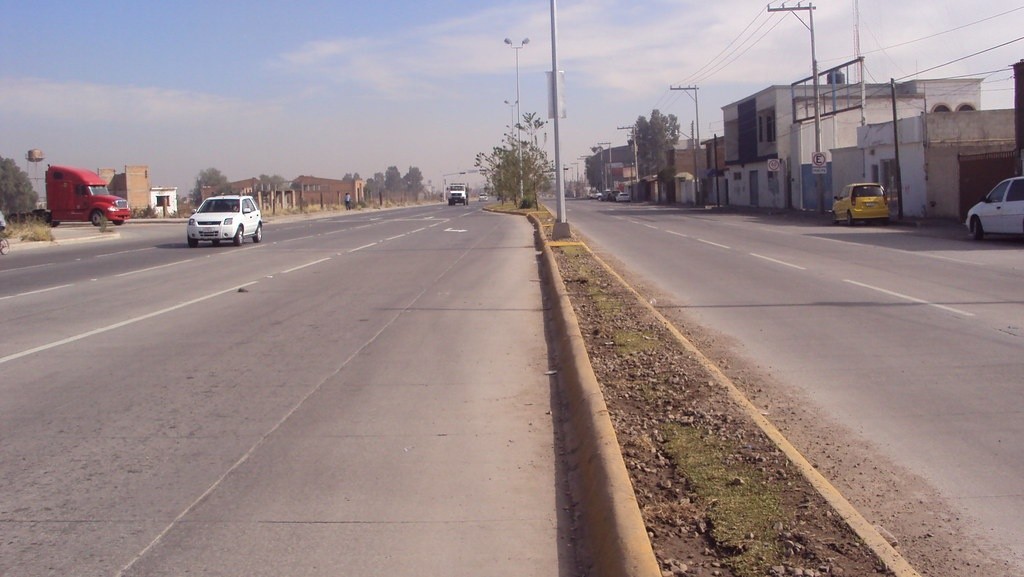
[447,182,469,206]
[479,192,488,202]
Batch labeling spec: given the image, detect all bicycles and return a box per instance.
[0,232,10,255]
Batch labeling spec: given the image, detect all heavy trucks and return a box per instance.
[5,164,132,227]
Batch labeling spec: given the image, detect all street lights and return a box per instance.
[504,37,530,198]
[504,100,519,150]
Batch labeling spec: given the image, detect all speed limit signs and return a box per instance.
[768,159,780,171]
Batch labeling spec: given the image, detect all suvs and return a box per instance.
[186,195,264,247]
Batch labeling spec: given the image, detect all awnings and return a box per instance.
[697,168,716,177]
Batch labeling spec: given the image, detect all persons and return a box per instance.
[344,193,351,210]
[0,210,6,249]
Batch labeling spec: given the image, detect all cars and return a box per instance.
[966,176,1024,240]
[498,193,506,201]
[832,183,890,226]
[587,191,631,202]
